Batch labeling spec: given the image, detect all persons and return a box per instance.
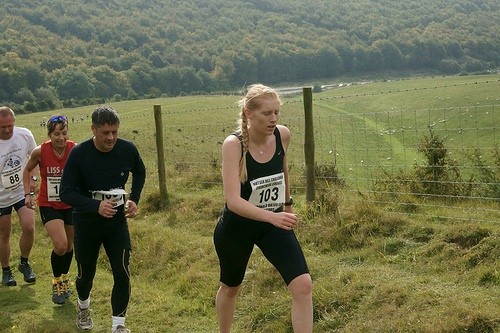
[58,106,147,333]
[23,115,78,305]
[213,83,314,333]
[0,106,40,287]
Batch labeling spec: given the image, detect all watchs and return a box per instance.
[30,175,38,182]
[25,192,34,197]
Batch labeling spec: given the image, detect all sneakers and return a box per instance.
[18,263,36,283]
[2,270,17,286]
[76,303,93,330]
[111,324,130,333]
[52,279,72,305]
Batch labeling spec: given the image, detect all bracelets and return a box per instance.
[285,196,293,206]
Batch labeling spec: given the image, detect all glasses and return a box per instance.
[47,115,67,123]
